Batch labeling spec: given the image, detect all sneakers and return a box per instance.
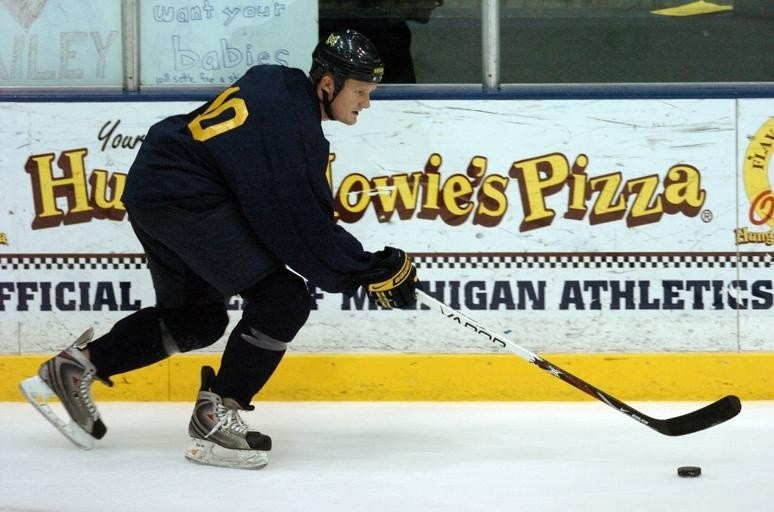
[190,366,271,450]
[39,328,106,439]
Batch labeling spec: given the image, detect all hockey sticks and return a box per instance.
[412,288,742,437]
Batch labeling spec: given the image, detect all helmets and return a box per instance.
[313,29,384,83]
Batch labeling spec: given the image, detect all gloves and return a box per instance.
[365,247,418,309]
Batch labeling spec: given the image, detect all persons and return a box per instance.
[38,28,419,450]
